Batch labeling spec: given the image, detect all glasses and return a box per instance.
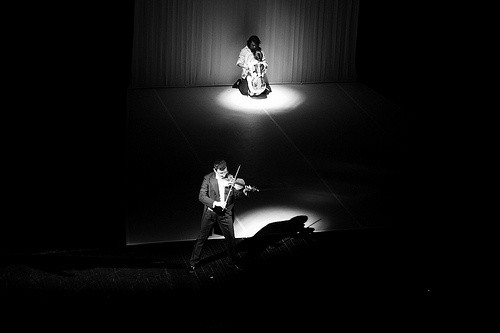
[216,171,228,176]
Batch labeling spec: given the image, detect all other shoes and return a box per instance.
[187,265,196,273]
[233,79,241,88]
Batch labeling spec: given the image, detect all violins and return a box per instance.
[222,173,261,192]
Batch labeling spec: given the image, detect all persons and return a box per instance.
[232,35,271,96]
[183,160,241,274]
